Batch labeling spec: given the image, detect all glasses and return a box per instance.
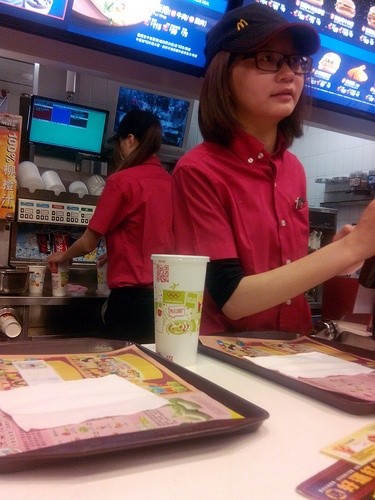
[228,50,313,74]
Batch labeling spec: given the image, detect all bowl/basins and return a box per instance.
[0,266,27,296]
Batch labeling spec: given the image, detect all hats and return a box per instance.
[203,3,320,56]
[105,109,158,143]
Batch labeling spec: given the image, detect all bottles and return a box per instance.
[346,170,375,191]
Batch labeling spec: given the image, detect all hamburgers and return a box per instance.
[318,52,341,74]
[335,0,356,18]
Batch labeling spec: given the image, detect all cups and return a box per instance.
[0,313,21,337]
[51,260,70,296]
[97,260,111,295]
[151,254,210,365]
[28,266,47,293]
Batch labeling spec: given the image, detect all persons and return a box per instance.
[170,4,375,335]
[46,108,172,343]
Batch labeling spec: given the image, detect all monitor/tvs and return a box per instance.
[112,84,194,163]
[27,94,110,161]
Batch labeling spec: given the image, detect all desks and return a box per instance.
[1,343,375,500]
